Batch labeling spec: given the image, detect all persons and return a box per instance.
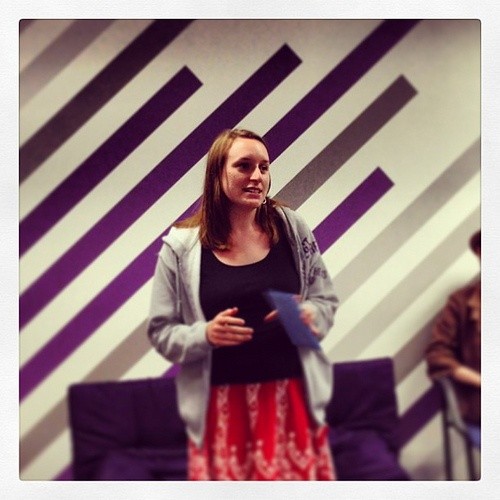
[423,230,482,427]
[146,129,340,481]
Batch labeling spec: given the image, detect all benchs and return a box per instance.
[56,358,405,482]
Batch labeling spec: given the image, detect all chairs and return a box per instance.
[433,372,481,480]
